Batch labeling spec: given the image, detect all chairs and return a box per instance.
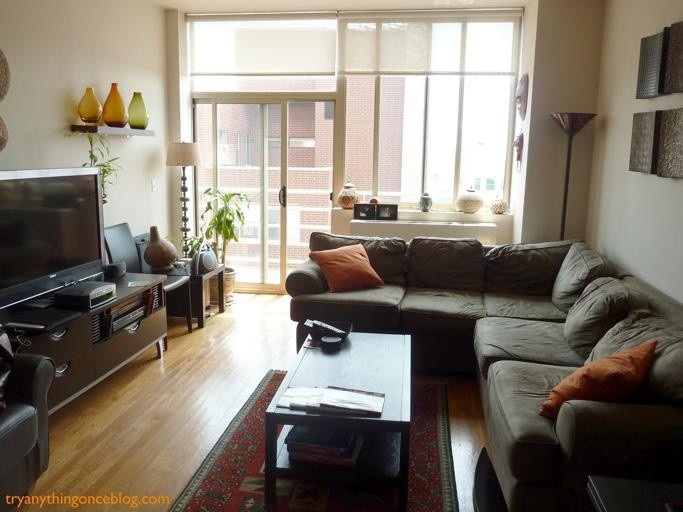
[103,222,192,351]
[0,353,56,511]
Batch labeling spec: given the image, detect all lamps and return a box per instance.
[165,142,203,258]
[545,111,598,242]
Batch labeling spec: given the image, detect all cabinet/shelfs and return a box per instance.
[0,271,168,418]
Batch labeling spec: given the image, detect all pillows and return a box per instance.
[309,242,385,293]
[537,337,659,422]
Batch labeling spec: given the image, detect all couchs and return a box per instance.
[284,230,682,512]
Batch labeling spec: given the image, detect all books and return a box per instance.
[284,424,363,467]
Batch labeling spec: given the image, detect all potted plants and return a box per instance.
[199,188,250,306]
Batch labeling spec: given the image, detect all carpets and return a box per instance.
[164,369,459,512]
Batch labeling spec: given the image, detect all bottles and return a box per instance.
[75,81,149,130]
[336,179,361,210]
[144,225,177,272]
[455,185,484,215]
[419,191,434,212]
[489,194,509,214]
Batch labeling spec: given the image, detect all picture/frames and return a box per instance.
[353,203,375,220]
[374,203,399,220]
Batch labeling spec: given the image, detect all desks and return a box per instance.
[585,475,683,512]
[164,263,226,329]
[348,220,498,245]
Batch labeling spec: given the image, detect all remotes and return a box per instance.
[6,320,47,332]
[313,321,346,336]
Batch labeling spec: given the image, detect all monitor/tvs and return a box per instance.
[0,167,106,309]
